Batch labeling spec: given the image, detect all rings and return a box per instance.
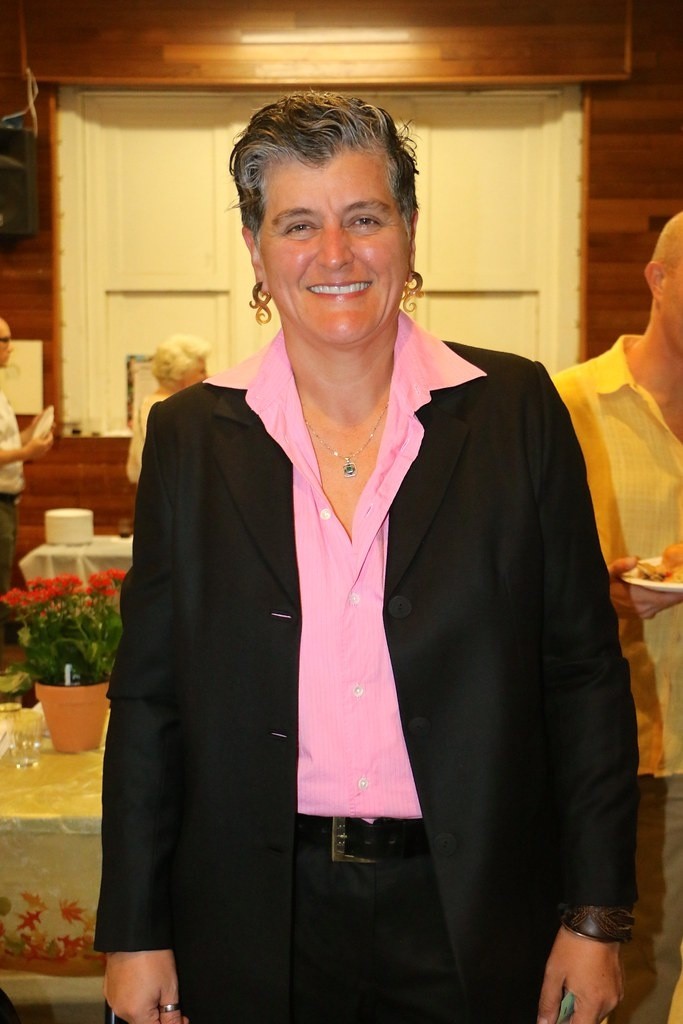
[158,1004,178,1013]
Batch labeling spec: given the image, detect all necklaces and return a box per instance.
[302,402,390,478]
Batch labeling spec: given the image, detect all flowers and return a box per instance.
[0,569,131,686]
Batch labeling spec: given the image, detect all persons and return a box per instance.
[1,314,52,635]
[550,200,683,1024]
[125,333,209,489]
[92,87,640,1024]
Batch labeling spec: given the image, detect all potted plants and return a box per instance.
[38,672,113,757]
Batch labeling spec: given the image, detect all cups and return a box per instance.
[5,708,42,770]
[118,518,131,539]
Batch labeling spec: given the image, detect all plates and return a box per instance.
[32,405,55,441]
[620,555,683,593]
[44,508,94,546]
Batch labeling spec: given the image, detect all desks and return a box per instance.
[1,689,121,1024]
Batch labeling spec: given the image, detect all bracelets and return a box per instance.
[558,906,634,945]
